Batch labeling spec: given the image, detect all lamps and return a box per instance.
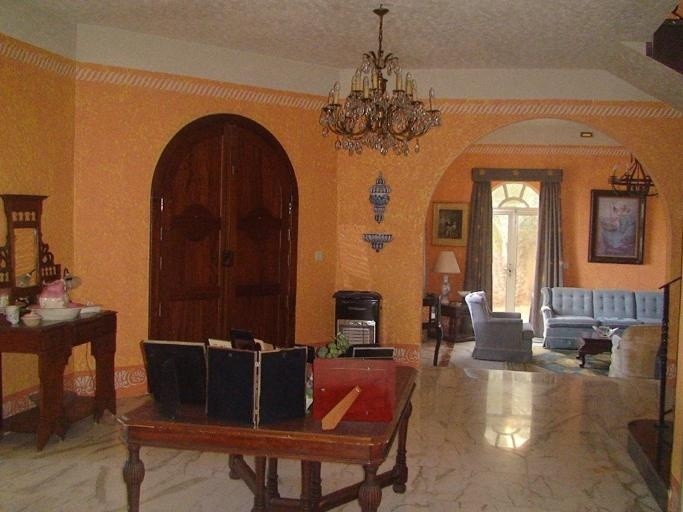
[15,265,36,288]
[314,2,443,158]
[432,249,461,305]
[607,151,658,200]
[59,264,79,293]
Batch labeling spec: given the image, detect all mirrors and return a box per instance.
[0,190,59,297]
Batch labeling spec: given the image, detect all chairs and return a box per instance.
[604,320,662,380]
[462,291,534,364]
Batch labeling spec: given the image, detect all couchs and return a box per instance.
[537,283,665,351]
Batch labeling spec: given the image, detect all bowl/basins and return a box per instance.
[28,308,81,320]
[20,311,42,327]
[456,291,471,297]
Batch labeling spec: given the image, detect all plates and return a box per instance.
[450,301,462,304]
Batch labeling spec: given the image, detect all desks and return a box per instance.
[422,295,446,367]
[0,301,119,457]
[428,302,474,341]
[116,364,421,512]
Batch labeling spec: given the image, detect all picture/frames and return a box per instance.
[138,338,206,421]
[311,356,399,432]
[429,201,469,248]
[585,188,648,267]
[202,344,307,429]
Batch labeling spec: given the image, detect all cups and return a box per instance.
[0,295,8,314]
[5,305,19,325]
[39,298,63,309]
